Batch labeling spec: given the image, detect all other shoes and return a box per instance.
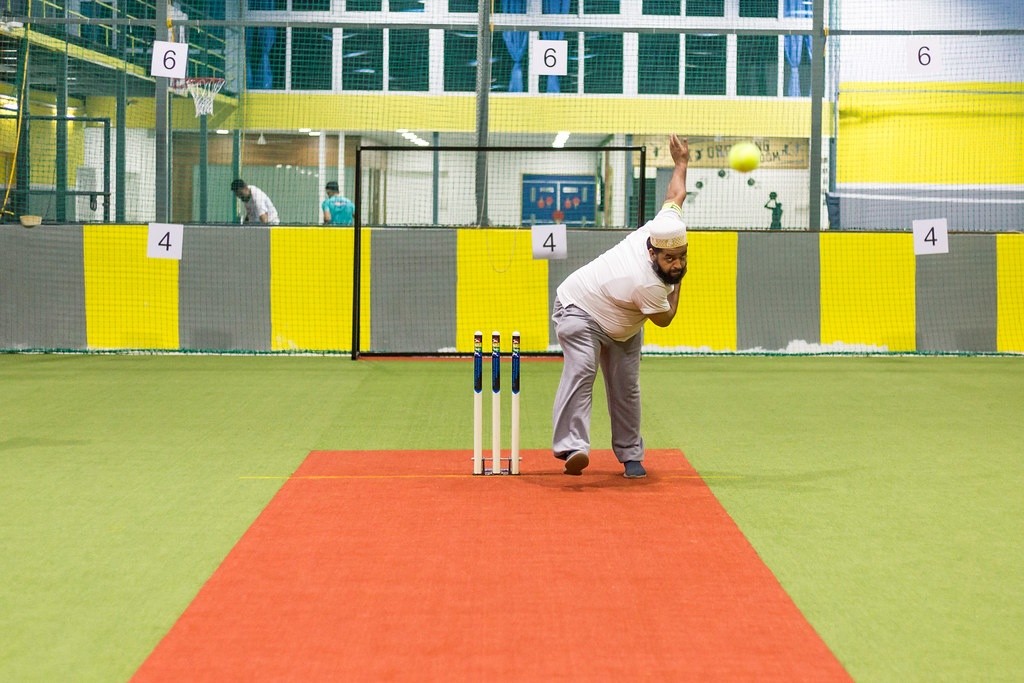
[564,451,590,475]
[624,461,646,478]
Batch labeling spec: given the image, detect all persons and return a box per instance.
[231,180,280,226]
[322,181,355,226]
[551,132,691,477]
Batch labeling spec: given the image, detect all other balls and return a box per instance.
[727,142,761,172]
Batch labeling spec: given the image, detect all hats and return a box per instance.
[650,219,688,248]
